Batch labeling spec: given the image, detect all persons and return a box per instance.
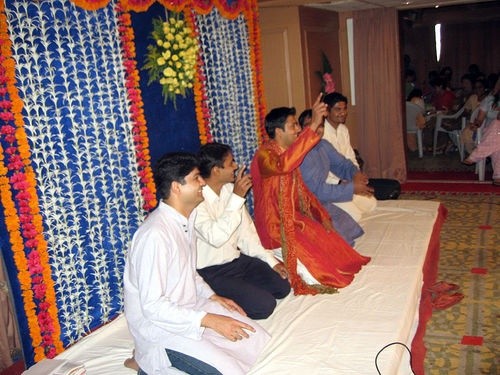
[297,108,374,248]
[322,92,377,224]
[252,92,371,296]
[190,143,291,320]
[404,55,500,184]
[124,152,271,375]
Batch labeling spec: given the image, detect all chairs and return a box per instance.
[433,106,488,182]
[407,102,424,159]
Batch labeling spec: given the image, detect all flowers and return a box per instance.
[140,7,201,111]
[314,48,336,125]
